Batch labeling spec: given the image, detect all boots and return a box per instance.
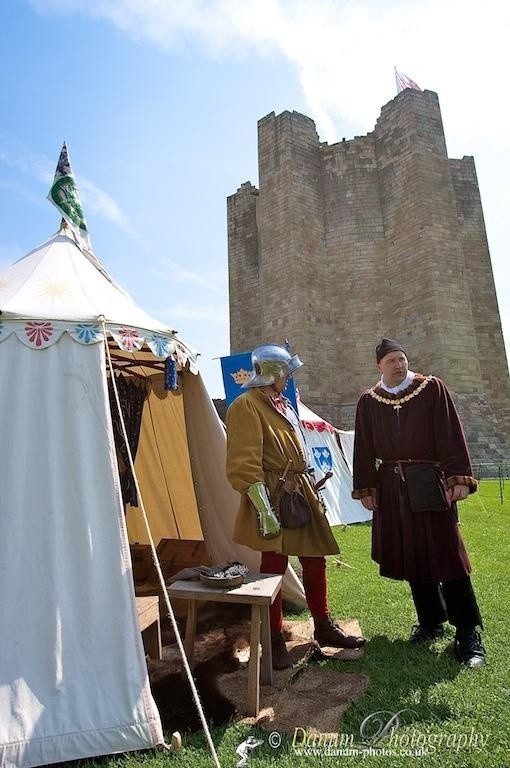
[272,632,292,670]
[313,617,366,648]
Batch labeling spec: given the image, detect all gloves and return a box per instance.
[246,482,281,540]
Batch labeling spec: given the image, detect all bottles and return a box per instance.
[164,353,179,391]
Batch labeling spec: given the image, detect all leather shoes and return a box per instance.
[410,624,445,645]
[456,627,485,668]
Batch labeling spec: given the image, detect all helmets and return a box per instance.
[241,344,303,389]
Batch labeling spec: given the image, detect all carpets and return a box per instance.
[143,613,369,742]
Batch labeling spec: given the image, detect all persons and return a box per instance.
[226,344,366,669]
[351,339,485,667]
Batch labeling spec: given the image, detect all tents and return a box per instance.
[0,141,307,768]
[295,387,374,528]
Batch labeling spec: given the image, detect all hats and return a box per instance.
[375,339,409,364]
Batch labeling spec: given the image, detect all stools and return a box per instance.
[136,596,162,660]
[168,573,283,718]
